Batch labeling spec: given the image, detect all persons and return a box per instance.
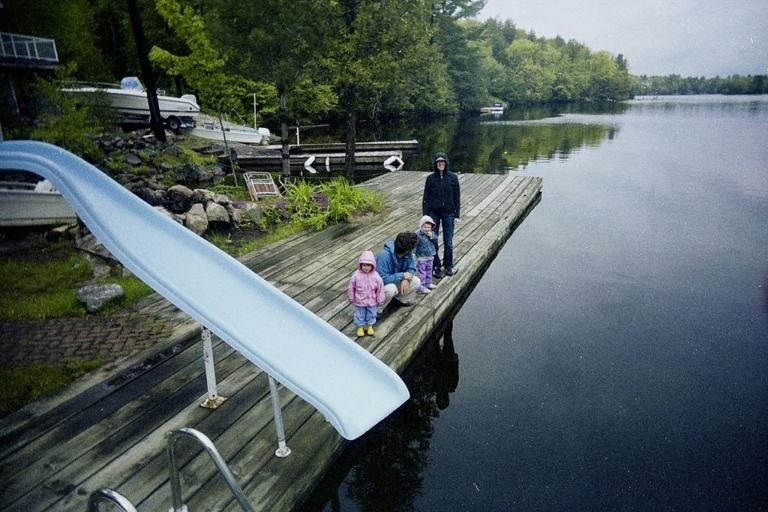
[413,214,438,293]
[423,152,460,277]
[375,231,421,319]
[348,251,385,336]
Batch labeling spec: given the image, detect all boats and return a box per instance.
[191,105,281,145]
[480,102,503,112]
[54,74,202,133]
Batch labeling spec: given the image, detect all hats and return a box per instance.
[436,156,445,161]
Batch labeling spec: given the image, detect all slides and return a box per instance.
[0,140,410,441]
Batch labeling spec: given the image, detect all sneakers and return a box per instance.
[418,287,431,294]
[392,297,411,307]
[445,266,453,276]
[367,326,375,335]
[376,313,382,320]
[432,265,443,278]
[356,326,365,336]
[428,283,437,289]
